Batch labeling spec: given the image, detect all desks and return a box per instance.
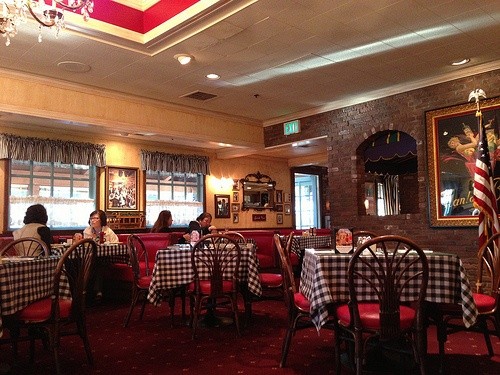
[148,242,261,323]
[0,255,74,361]
[300,246,479,375]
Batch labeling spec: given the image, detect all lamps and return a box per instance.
[173,53,195,68]
[0,0,96,47]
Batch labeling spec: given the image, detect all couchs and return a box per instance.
[282,229,332,247]
[116,232,178,297]
[218,229,281,296]
[51,241,136,299]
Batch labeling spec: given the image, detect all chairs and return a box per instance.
[0,237,53,354]
[10,236,98,375]
[435,232,500,360]
[272,233,339,368]
[188,233,241,339]
[259,231,295,324]
[352,231,386,248]
[337,235,428,375]
[123,234,186,330]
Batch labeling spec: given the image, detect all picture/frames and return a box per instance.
[275,190,283,204]
[276,214,284,224]
[284,204,291,215]
[233,213,239,223]
[214,194,231,219]
[275,205,283,212]
[231,204,240,212]
[233,192,239,202]
[232,179,240,191]
[424,97,500,230]
[104,165,142,213]
[252,214,267,222]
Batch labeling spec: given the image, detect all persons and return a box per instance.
[189,213,216,244]
[17,204,51,256]
[151,210,190,243]
[83,210,119,297]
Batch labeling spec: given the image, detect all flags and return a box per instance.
[472,115,500,288]
